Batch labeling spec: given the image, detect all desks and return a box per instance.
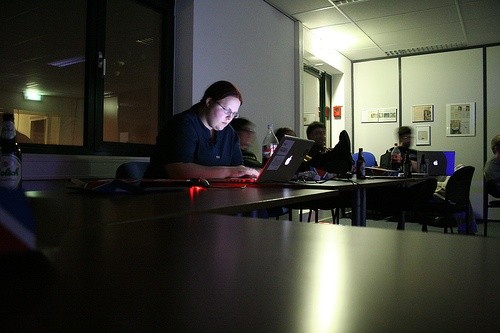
[0,176,500,333]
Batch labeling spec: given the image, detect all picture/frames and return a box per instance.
[416,126,431,146]
[412,104,434,123]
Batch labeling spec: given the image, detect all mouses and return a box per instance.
[191,178,209,188]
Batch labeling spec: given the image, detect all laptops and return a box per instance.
[206,135,315,183]
[417,150,455,176]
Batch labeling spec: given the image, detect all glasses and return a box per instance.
[236,128,255,134]
[216,101,240,118]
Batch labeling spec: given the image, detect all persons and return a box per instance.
[484,135,500,198]
[385,126,419,221]
[231,117,355,174]
[143,80,261,179]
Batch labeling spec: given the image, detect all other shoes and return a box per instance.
[333,130,355,174]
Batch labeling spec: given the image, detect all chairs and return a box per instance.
[422,166,475,233]
[483,175,500,237]
[352,152,377,169]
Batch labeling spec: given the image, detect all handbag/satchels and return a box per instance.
[435,164,465,194]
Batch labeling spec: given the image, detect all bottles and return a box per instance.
[356,149,365,180]
[391,143,402,173]
[420,154,427,174]
[0,113,22,191]
[404,155,413,178]
[262,124,279,170]
